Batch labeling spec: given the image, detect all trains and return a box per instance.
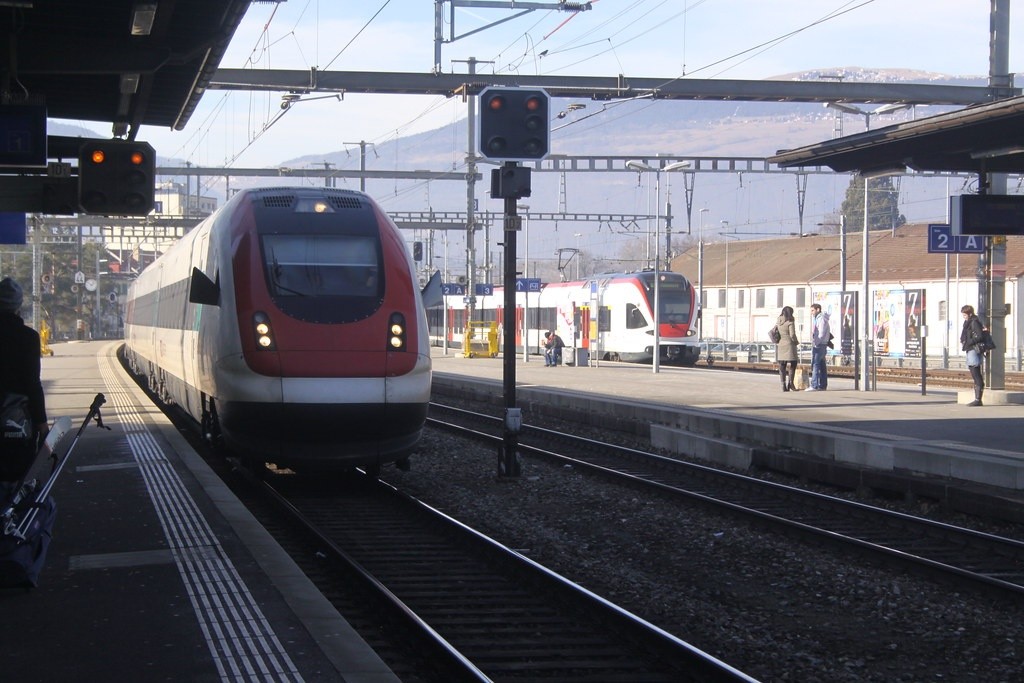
[419,269,702,366]
[122,185,432,485]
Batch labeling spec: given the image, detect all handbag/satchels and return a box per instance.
[769,325,781,344]
[0,393,33,442]
[793,368,809,390]
[971,321,997,354]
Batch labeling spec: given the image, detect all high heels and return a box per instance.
[787,381,796,390]
[781,382,787,392]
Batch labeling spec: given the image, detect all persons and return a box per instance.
[78,328,82,340]
[960,305,984,406]
[88,323,93,339]
[776,306,799,391]
[0,278,49,500]
[542,332,565,367]
[805,304,830,391]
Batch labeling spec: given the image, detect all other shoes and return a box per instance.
[544,364,550,366]
[550,363,557,367]
[805,386,817,391]
[967,400,983,406]
[816,386,826,391]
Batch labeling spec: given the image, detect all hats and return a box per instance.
[544,332,551,338]
[0,277,23,311]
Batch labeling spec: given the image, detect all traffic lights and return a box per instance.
[78,137,155,216]
[413,241,423,261]
[477,85,551,162]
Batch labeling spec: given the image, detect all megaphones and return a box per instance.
[458,275,466,285]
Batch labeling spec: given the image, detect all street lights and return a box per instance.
[485,189,491,213]
[822,101,912,392]
[516,203,528,363]
[574,234,582,280]
[816,215,847,291]
[626,159,692,373]
[720,220,729,342]
[697,207,710,341]
